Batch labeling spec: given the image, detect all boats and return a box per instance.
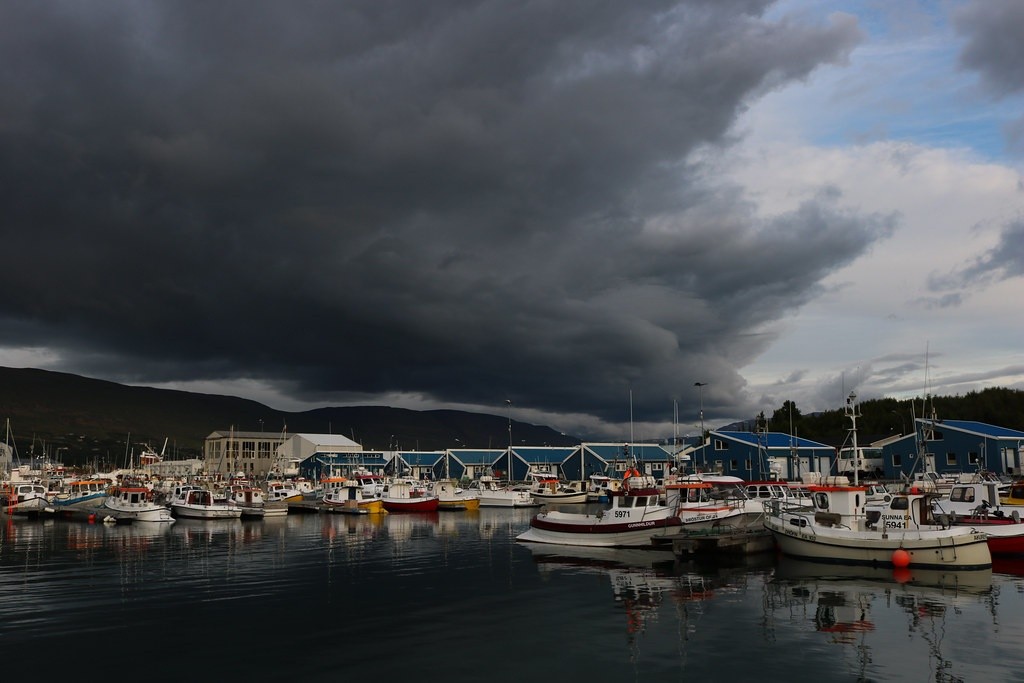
[0,420,1024,591]
[761,486,993,588]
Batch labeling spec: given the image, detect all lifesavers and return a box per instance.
[624,469,640,480]
